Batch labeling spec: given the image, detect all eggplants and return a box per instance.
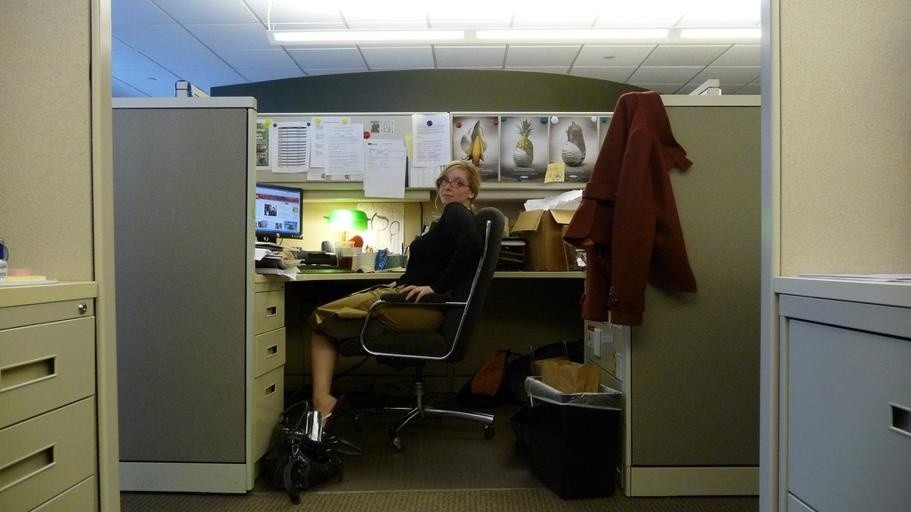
[567,121,586,160]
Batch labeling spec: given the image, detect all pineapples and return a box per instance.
[513,138,533,166]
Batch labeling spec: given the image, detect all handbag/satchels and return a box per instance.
[266,400,361,508]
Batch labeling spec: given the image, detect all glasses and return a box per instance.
[438,177,471,188]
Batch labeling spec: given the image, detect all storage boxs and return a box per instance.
[511,206,584,273]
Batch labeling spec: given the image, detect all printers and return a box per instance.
[299,250,337,267]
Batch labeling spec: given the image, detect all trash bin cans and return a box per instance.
[525,375,624,501]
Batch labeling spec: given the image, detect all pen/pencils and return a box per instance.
[401,243,408,256]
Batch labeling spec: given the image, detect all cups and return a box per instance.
[387,255,408,269]
[335,239,377,272]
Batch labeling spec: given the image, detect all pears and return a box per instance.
[562,141,582,166]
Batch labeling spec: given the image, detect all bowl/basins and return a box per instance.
[283,258,304,269]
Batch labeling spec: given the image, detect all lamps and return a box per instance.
[321,207,389,234]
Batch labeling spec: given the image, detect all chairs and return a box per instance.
[341,206,504,454]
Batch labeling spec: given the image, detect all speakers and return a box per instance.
[321,241,335,253]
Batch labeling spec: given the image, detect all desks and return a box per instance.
[253,265,623,484]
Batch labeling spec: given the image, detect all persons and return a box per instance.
[304,161,484,431]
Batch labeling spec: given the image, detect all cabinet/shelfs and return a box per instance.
[0,282,101,512]
[775,275,911,512]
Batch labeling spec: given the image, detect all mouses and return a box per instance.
[356,269,363,273]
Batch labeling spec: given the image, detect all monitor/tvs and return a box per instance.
[255,182,304,246]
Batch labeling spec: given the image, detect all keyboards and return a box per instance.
[301,268,352,274]
